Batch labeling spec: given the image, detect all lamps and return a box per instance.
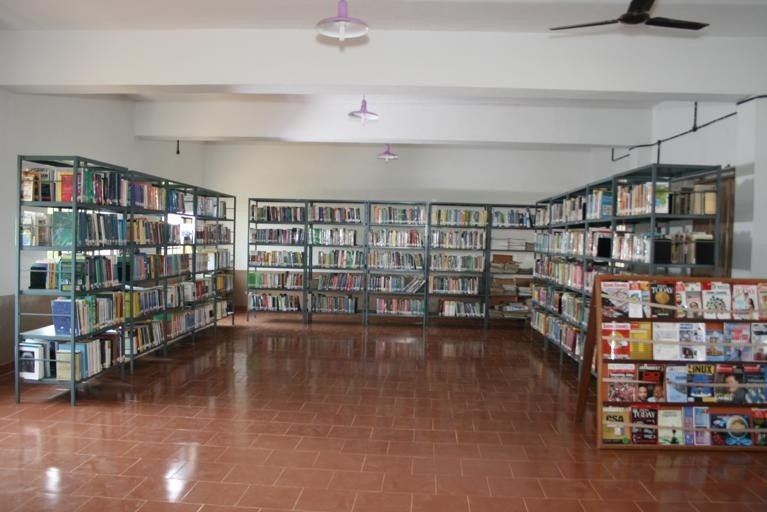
[349,95,378,125]
[313,1,370,43]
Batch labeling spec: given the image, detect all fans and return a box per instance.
[549,1,712,31]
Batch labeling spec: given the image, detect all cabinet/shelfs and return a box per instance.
[245,199,543,330]
[530,162,767,453]
[17,155,237,408]
